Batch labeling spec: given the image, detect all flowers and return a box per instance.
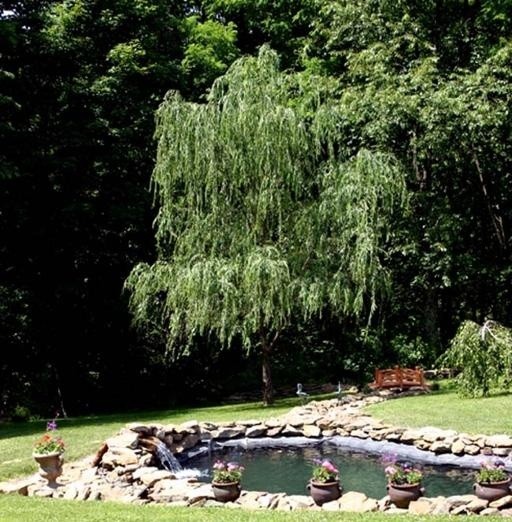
[310,456,340,483]
[475,458,509,482]
[209,458,244,481]
[382,446,427,483]
[32,411,66,453]
